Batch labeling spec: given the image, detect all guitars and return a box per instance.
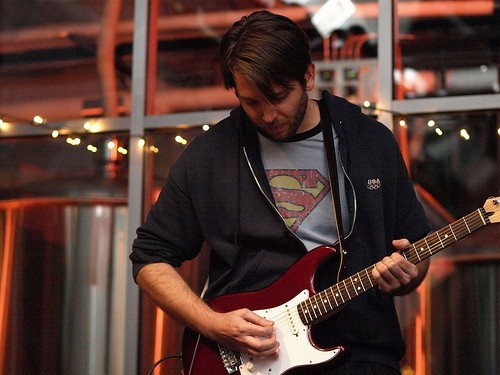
[179,194,500,375]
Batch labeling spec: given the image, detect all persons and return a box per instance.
[128,11,431,375]
